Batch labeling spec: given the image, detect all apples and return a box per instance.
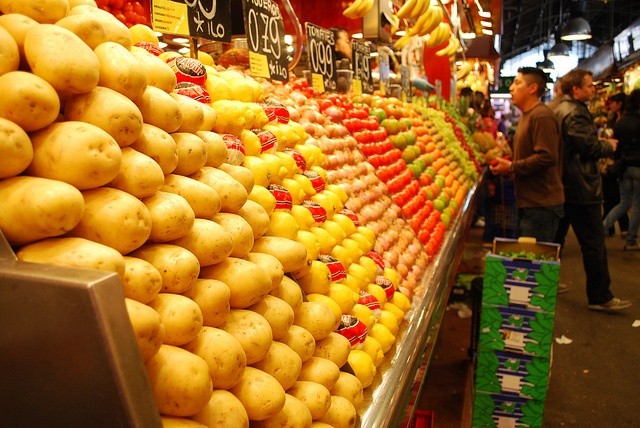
[320,92,466,264]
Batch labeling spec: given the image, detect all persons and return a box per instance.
[547,77,563,109]
[498,114,507,133]
[601,89,640,250]
[602,92,630,237]
[488,67,566,242]
[553,68,633,311]
[460,87,488,116]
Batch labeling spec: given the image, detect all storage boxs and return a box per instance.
[475,347,550,401]
[482,236,561,313]
[471,391,546,428]
[480,304,556,359]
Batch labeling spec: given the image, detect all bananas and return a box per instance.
[395,0,430,21]
[427,22,451,46]
[436,34,460,57]
[410,6,444,35]
[343,0,373,19]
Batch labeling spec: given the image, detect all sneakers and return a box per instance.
[587,296,633,310]
[557,280,570,293]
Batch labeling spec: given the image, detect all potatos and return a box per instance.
[0,0,362,427]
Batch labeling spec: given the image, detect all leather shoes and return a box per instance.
[624,244,640,251]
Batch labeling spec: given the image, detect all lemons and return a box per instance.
[142,24,409,389]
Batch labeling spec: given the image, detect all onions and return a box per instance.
[263,83,428,297]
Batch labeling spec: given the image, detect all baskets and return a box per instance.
[494,204,517,229]
